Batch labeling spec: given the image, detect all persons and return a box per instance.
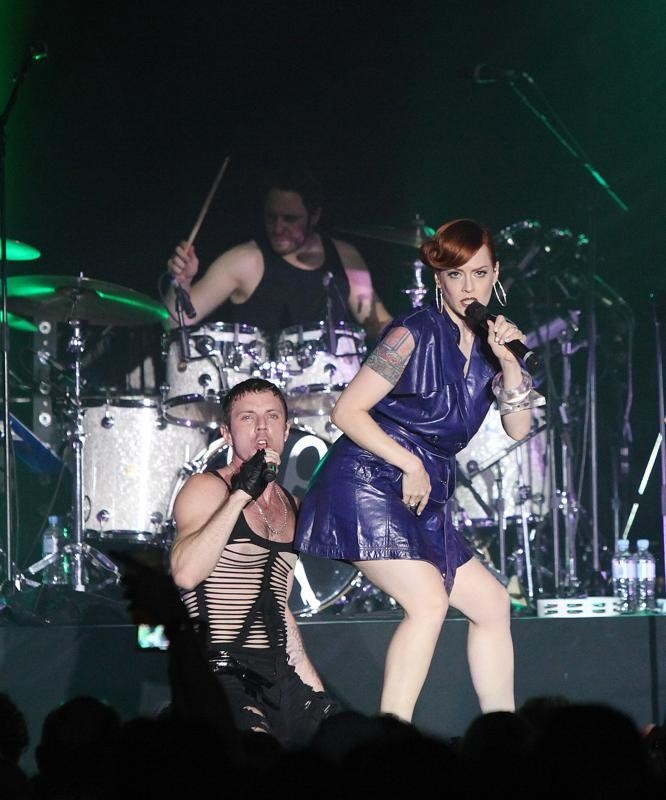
[291,221,534,725]
[169,379,344,747]
[0,688,666,800]
[161,172,394,359]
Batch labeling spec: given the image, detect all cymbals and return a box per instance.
[1,274,172,326]
[536,596,624,619]
[339,222,439,249]
[0,237,41,261]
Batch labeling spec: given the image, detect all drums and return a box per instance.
[166,427,362,617]
[68,389,214,548]
[159,321,271,429]
[294,411,346,446]
[272,319,370,419]
[450,388,554,529]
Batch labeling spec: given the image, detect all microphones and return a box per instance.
[263,446,277,482]
[474,64,528,85]
[464,301,539,369]
[172,277,195,319]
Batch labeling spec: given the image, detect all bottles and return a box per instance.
[611,539,638,615]
[620,576,621,577]
[42,516,64,584]
[64,528,76,591]
[633,539,656,611]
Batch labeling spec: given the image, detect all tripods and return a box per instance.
[0,238,124,608]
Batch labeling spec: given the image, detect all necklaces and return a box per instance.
[255,483,287,534]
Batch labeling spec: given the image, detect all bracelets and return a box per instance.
[490,367,547,417]
